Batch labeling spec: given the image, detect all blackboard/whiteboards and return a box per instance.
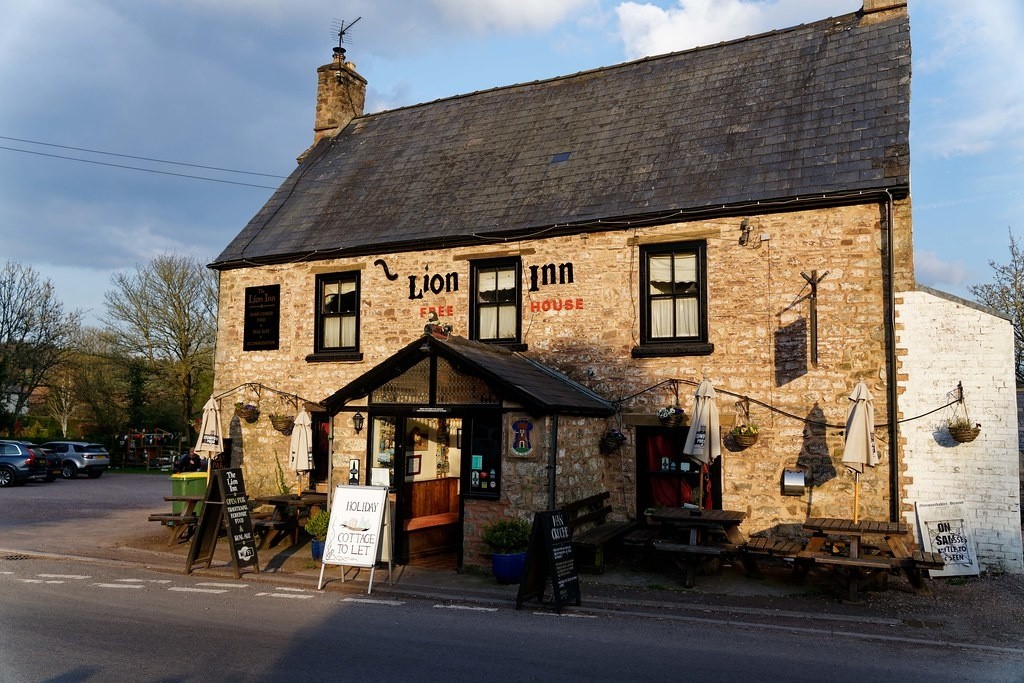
[185,468,259,568]
[323,485,392,567]
[516,510,582,602]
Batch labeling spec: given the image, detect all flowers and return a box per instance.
[659,415,684,430]
[658,406,685,420]
[268,413,294,432]
[729,422,760,436]
[601,428,627,452]
[235,402,254,418]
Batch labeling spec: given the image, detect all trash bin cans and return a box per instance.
[168,472,207,525]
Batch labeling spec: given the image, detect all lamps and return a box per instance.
[353,409,365,434]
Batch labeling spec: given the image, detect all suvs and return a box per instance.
[41,448,62,482]
[39,442,109,480]
[0,439,48,488]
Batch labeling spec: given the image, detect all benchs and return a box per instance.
[150,511,197,538]
[148,516,196,546]
[745,537,776,566]
[653,540,725,573]
[624,528,651,563]
[772,538,945,606]
[252,518,291,550]
[562,491,629,575]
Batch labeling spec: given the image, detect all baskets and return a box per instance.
[732,403,759,447]
[599,415,624,451]
[948,397,981,444]
[659,392,684,431]
[235,390,255,419]
[271,396,294,430]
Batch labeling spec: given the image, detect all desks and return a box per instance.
[802,517,908,596]
[253,494,327,519]
[651,506,746,566]
[162,495,205,541]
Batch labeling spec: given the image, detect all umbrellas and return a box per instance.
[194,394,224,487]
[289,406,315,545]
[683,374,721,511]
[841,375,879,525]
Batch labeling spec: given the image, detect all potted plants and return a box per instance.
[305,510,330,560]
[948,420,981,444]
[481,516,532,585]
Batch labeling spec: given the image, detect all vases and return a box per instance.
[235,408,253,418]
[272,420,291,431]
[732,434,759,447]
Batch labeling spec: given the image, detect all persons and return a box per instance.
[200,456,207,470]
[174,447,203,474]
